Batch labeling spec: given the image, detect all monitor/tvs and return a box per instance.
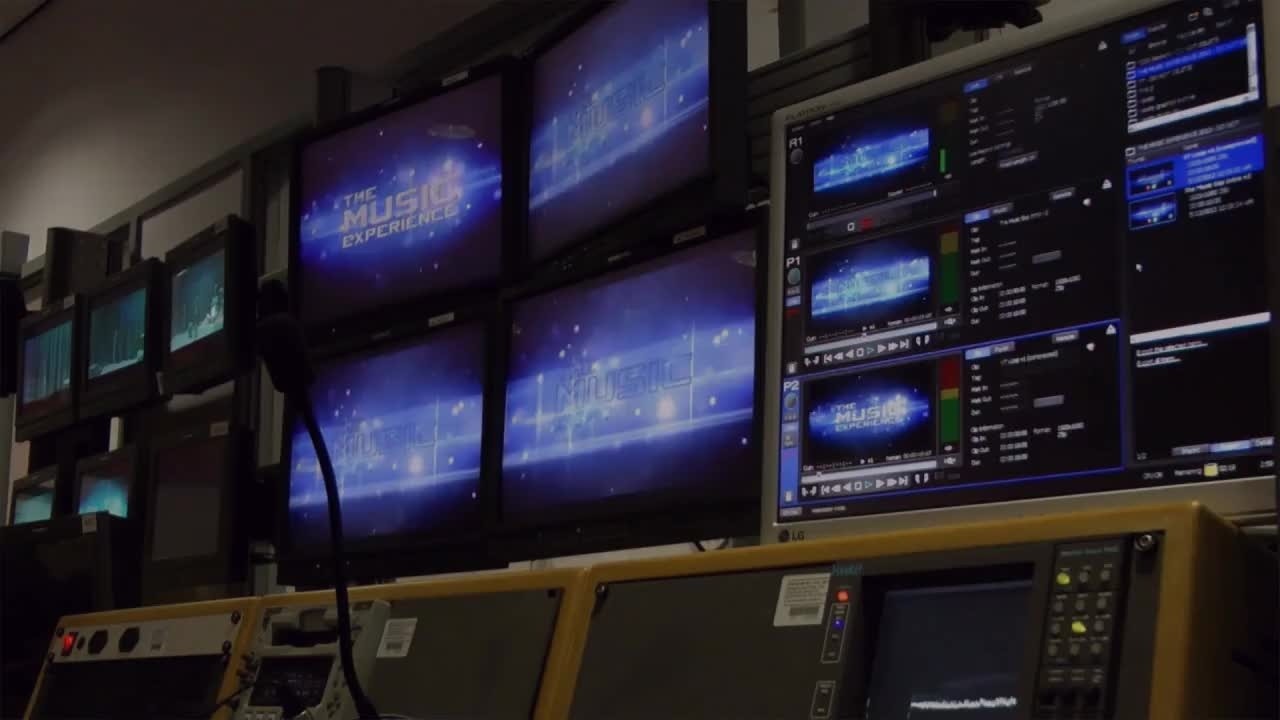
[513,0,750,276]
[858,560,1042,720]
[10,213,250,580]
[777,1,1280,526]
[291,54,513,345]
[277,290,501,577]
[494,204,768,541]
